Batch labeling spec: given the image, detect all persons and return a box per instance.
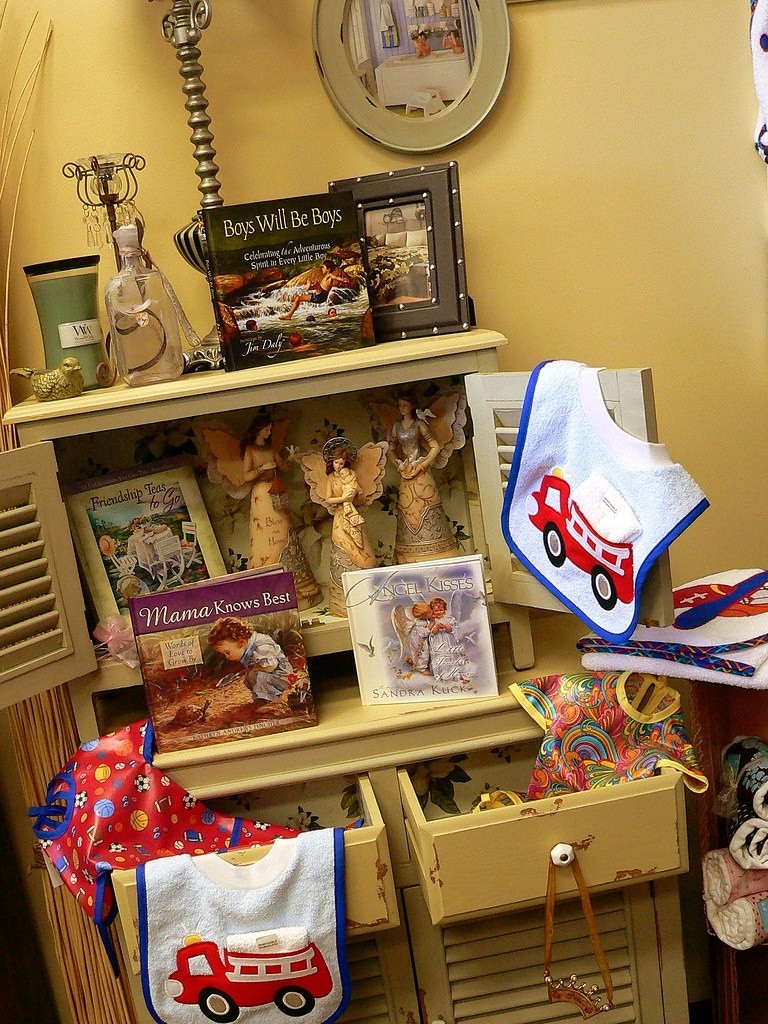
[278,260,348,320]
[241,415,324,611]
[328,308,336,317]
[288,333,301,347]
[246,320,257,330]
[324,447,379,616]
[386,394,460,564]
[306,316,316,321]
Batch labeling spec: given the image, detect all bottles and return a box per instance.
[104,224,184,388]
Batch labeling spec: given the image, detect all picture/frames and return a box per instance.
[328,160,471,343]
[311,0,511,155]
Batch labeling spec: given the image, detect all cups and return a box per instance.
[22,254,114,391]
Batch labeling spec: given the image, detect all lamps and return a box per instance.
[62,153,167,376]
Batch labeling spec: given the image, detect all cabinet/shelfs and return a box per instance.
[0,331,692,1024]
